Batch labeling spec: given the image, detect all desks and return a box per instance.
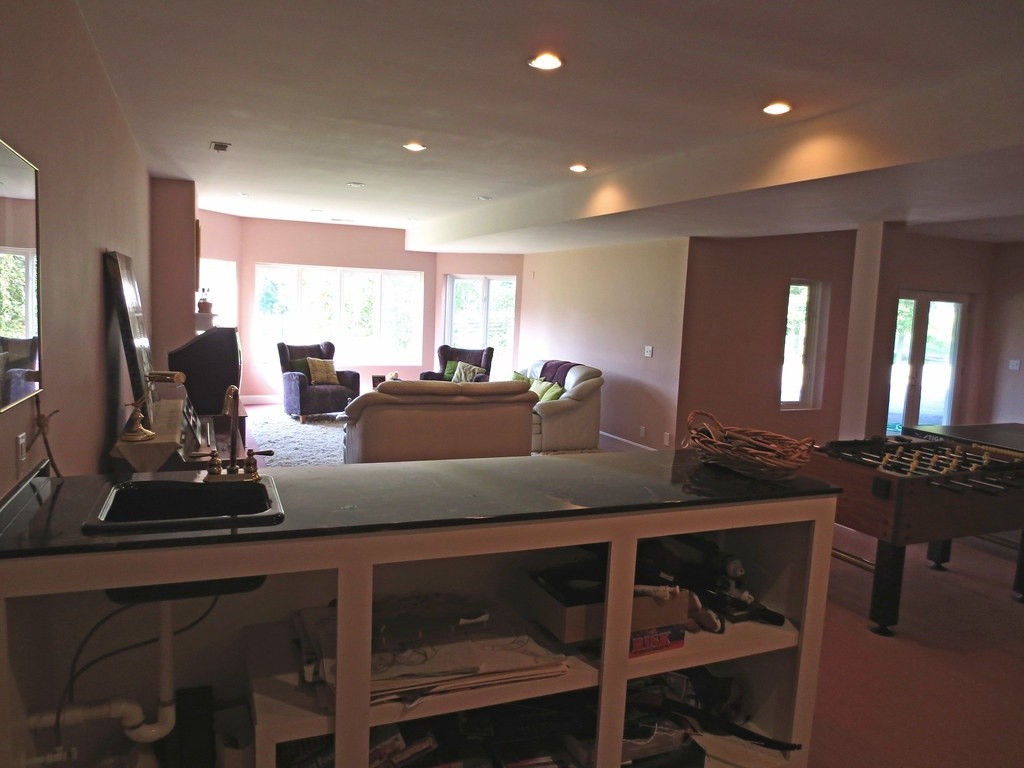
[903,422,1024,598]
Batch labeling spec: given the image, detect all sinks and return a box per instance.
[81,476,285,604]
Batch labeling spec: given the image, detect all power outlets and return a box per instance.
[640,426,646,438]
[664,432,670,447]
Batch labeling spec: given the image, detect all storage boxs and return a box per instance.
[519,565,689,644]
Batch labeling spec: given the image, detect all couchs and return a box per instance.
[277,341,360,424]
[335,381,538,464]
[518,360,604,451]
[421,345,494,383]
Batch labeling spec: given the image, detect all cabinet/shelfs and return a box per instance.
[2,450,841,768]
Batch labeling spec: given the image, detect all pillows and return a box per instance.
[442,360,459,382]
[307,357,340,385]
[290,358,313,384]
[452,360,486,383]
[512,370,545,386]
[541,382,564,402]
[528,379,553,402]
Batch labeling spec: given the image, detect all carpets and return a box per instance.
[250,413,346,469]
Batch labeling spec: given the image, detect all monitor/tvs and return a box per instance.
[168,327,242,416]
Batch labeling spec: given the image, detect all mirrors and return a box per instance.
[0,139,44,414]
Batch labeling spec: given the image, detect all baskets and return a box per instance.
[686,409,816,481]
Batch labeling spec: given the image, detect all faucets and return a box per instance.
[222,384,239,474]
